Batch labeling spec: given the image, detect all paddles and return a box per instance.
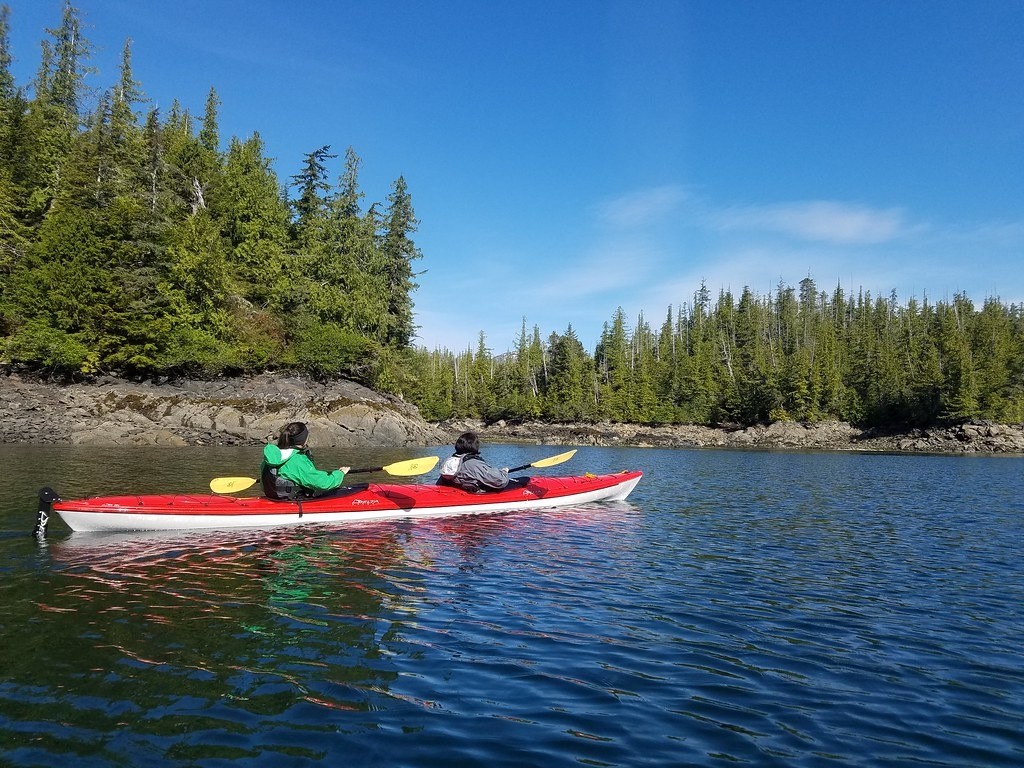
[504,448,578,473]
[209,455,441,498]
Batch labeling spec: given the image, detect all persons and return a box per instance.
[260,422,368,502]
[438,432,530,496]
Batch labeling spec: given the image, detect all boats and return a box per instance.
[38,470,644,532]
